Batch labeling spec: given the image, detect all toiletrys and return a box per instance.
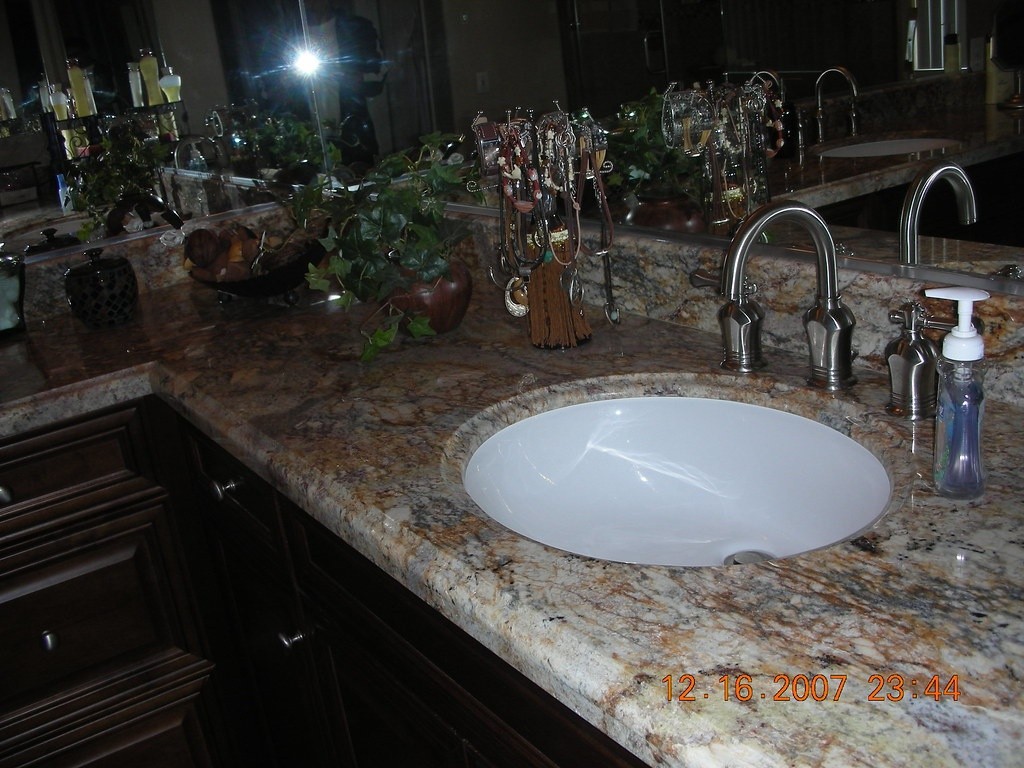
[924,286,991,502]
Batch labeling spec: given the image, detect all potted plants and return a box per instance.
[251,129,476,365]
[250,111,367,198]
[601,89,706,235]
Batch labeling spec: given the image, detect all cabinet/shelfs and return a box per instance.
[173,412,650,768]
[0,404,277,768]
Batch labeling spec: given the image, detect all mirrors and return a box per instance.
[0,0,1024,296]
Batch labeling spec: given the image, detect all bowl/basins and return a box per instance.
[183,209,331,299]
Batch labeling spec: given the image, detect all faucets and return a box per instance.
[716,206,858,393]
[899,158,977,265]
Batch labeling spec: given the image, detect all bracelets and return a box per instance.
[660,93,694,150]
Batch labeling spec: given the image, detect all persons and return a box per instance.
[266,0,384,168]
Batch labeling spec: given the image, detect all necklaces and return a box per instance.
[682,78,786,246]
[495,117,619,349]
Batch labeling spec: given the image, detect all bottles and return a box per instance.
[39,80,52,115]
[66,58,98,118]
[49,82,68,122]
[160,67,182,104]
[128,62,144,107]
[63,88,78,119]
[944,34,959,74]
[140,48,164,106]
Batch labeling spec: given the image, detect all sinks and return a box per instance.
[439,369,919,571]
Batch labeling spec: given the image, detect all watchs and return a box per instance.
[474,122,501,177]
